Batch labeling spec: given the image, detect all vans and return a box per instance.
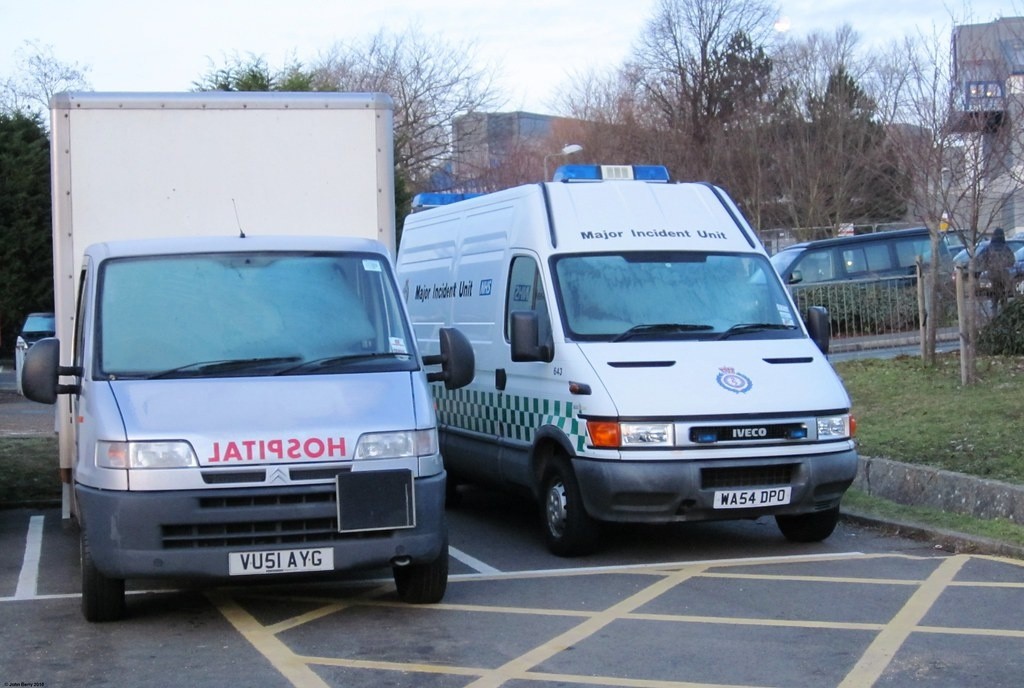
[739,226,954,314]
[395,178,860,559]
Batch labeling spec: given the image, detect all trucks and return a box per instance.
[20,90,475,622]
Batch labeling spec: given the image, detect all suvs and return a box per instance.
[15,313,56,370]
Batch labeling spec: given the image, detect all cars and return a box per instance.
[977,245,1023,296]
[951,238,1024,280]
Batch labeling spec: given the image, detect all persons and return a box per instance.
[982,228,1015,316]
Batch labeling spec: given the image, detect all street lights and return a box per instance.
[941,167,951,210]
[544,145,584,182]
